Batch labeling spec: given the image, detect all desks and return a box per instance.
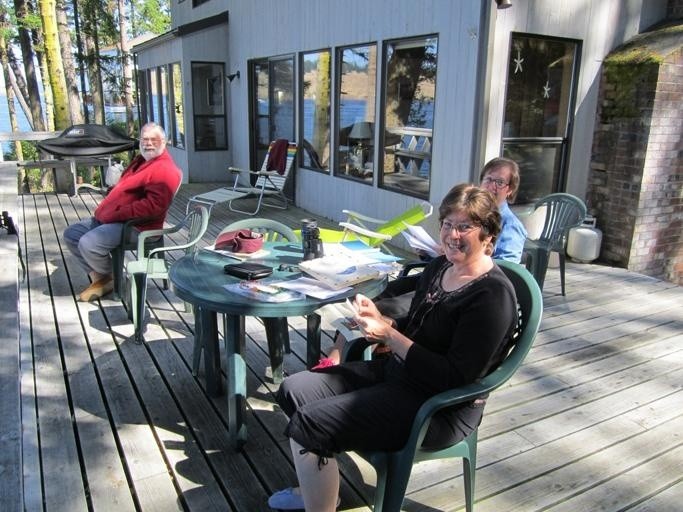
[168,242,388,450]
[341,170,430,200]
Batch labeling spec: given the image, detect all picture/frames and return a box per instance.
[206,77,222,106]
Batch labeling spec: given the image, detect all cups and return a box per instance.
[300,216,325,261]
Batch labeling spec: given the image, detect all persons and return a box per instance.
[309,156,526,370]
[268,181,517,512]
[63,122,180,301]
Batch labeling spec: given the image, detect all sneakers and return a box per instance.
[268,486,343,509]
[79,279,114,301]
[310,357,338,370]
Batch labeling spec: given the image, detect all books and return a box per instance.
[297,253,378,290]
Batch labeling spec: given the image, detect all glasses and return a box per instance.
[483,175,511,189]
[438,220,482,233]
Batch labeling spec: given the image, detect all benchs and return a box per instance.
[0,157,111,512]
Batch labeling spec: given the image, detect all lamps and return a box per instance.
[225,70,240,83]
[348,122,374,169]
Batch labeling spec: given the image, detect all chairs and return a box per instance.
[126,205,209,344]
[395,235,539,280]
[111,170,183,299]
[339,259,545,512]
[518,193,587,296]
[187,140,300,226]
[256,200,433,256]
[212,217,301,354]
